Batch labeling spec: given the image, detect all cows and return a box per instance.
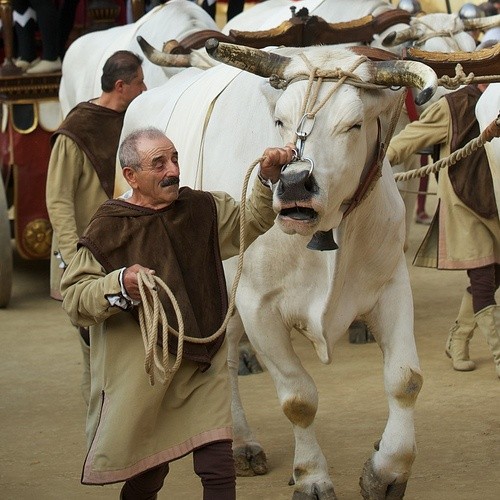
[475,83,500,223]
[112,37,438,500]
[223,0,500,53]
[59,0,287,120]
[387,79,470,254]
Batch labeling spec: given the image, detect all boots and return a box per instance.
[445,286,500,378]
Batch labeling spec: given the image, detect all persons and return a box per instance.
[385,39,500,380]
[0,0,80,74]
[45,50,147,304]
[58,127,297,500]
[466,0,500,48]
